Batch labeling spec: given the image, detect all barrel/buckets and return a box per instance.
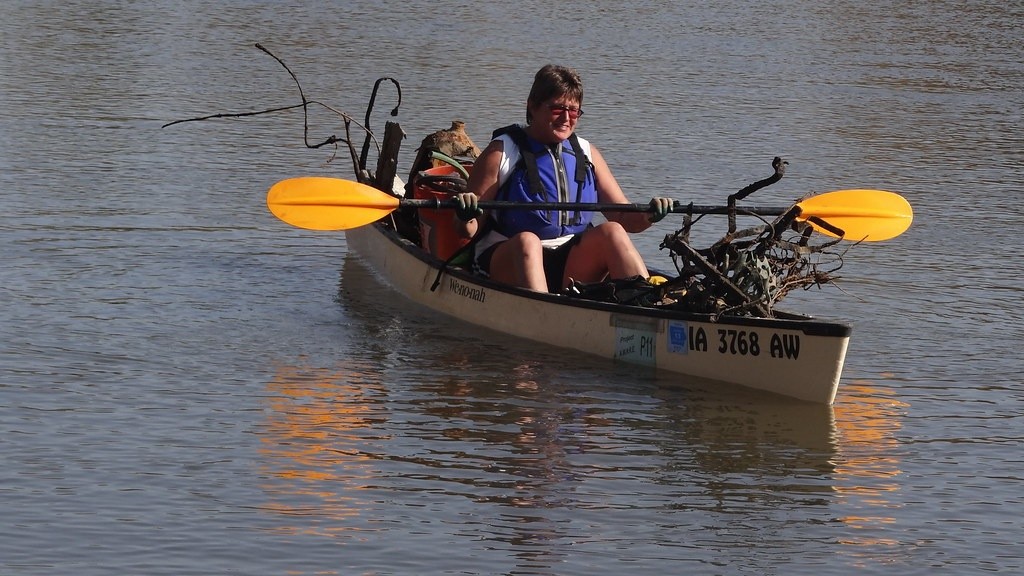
[413,166,483,266]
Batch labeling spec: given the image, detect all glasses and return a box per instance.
[537,101,583,119]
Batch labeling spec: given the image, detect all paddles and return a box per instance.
[267,175,916,243]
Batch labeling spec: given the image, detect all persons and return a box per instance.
[452,64,674,300]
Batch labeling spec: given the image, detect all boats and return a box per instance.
[345,168,853,406]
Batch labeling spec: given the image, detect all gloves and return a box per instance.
[453,192,483,222]
[647,197,673,222]
